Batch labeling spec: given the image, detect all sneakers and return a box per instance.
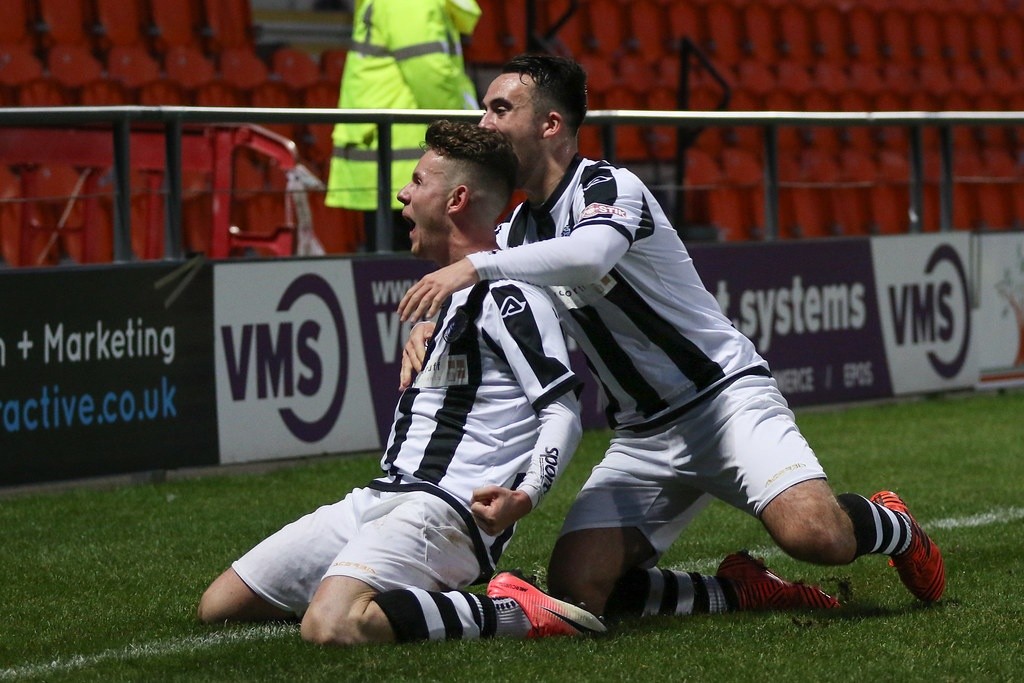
[486,569,609,639]
[870,491,944,602]
[716,550,841,610]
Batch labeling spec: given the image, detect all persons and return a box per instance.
[198,121,610,647]
[397,49,946,621]
[325,0,481,255]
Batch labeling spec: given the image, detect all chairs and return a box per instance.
[467,0,1024,237]
[0,0,365,263]
